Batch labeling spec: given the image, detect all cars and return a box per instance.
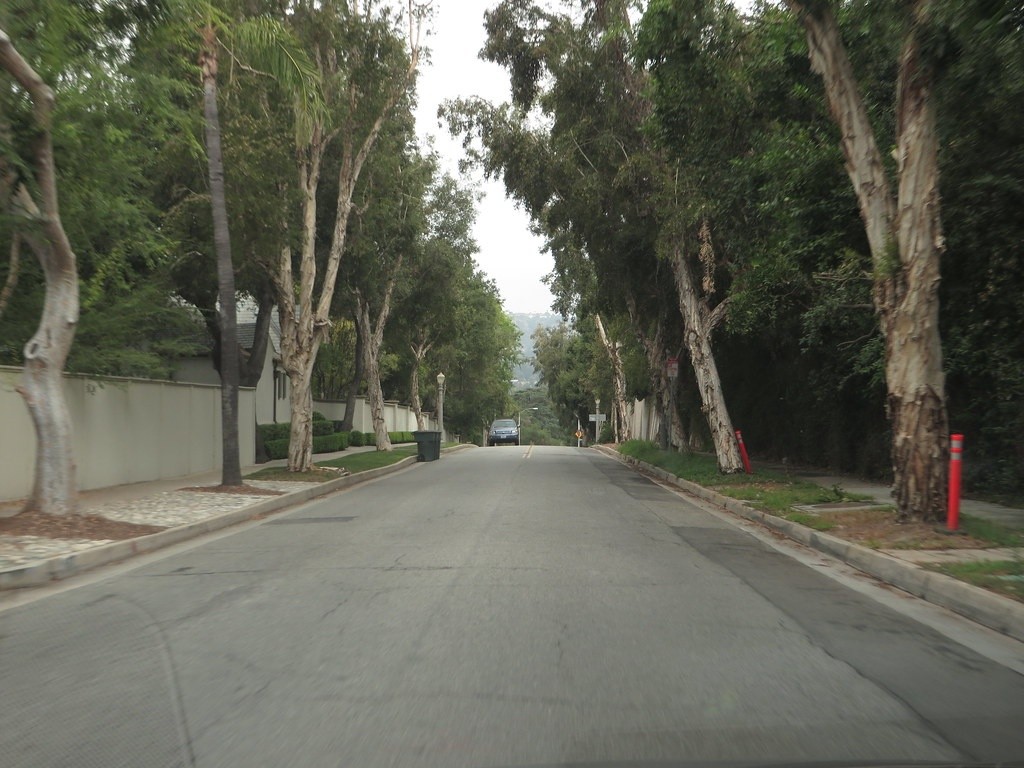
[487,419,520,446]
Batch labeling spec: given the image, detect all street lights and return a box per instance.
[595,399,600,442]
[437,372,445,442]
[519,408,538,445]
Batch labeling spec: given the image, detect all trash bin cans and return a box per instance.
[411,430,442,462]
